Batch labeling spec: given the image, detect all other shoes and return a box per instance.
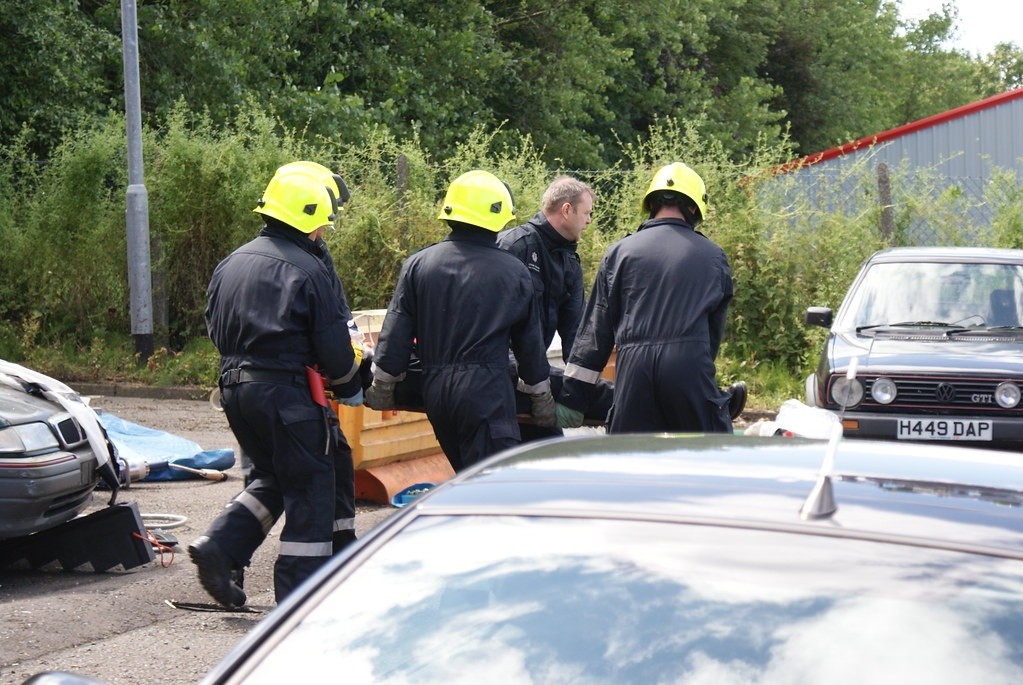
[188,536,246,607]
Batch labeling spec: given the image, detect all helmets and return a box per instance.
[437,170,517,232]
[253,169,338,234]
[275,161,352,210]
[641,162,708,221]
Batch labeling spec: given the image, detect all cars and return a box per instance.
[0,357,132,544]
[23,428,1022,685]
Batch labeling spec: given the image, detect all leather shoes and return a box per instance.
[727,380,748,421]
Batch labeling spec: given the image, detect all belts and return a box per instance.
[224,369,307,388]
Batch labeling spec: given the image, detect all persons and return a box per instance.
[555,161,733,435]
[347,342,747,420]
[494,174,597,365]
[367,170,556,474]
[184,160,364,607]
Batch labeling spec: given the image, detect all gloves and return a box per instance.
[332,388,364,407]
[557,403,584,428]
[366,377,395,411]
[529,392,557,428]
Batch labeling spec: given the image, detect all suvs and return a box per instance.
[805,245,1023,450]
